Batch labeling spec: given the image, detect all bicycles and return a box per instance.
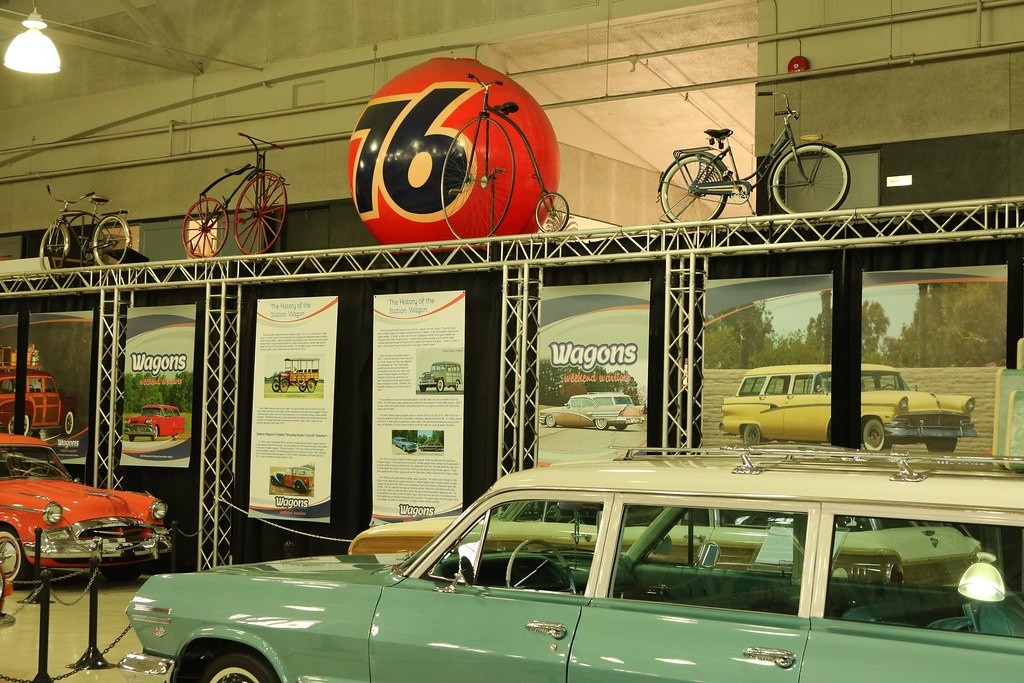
[656,90,852,223]
[39,184,132,269]
[440,71,570,240]
[182,131,288,257]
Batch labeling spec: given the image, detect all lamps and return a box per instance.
[4,0,62,75]
[957,552,1024,611]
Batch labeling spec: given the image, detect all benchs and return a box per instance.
[690,585,963,631]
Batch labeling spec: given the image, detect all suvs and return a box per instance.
[417,361,463,392]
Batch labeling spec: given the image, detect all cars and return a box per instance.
[392,436,417,451]
[0,431,174,583]
[419,443,443,451]
[0,361,78,436]
[347,500,988,589]
[124,404,185,441]
[719,362,977,453]
[123,448,1024,681]
[539,391,645,432]
[271,357,320,391]
[271,466,314,493]
